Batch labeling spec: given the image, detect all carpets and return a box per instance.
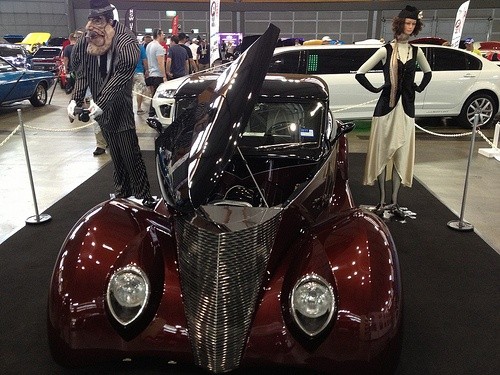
[0,149,500,375]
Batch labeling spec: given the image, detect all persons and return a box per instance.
[58,27,233,155]
[67,0,152,201]
[465,37,482,56]
[322,36,331,45]
[355,5,432,206]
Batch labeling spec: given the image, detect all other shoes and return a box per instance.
[137,110,145,114]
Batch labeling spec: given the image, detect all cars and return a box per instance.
[46,23,406,375]
[232,35,500,62]
[150,43,500,132]
[0,32,76,107]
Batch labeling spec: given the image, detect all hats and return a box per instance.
[398,5,423,21]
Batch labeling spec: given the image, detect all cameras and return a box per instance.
[73,107,91,123]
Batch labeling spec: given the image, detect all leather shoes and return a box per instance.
[93,147,105,154]
